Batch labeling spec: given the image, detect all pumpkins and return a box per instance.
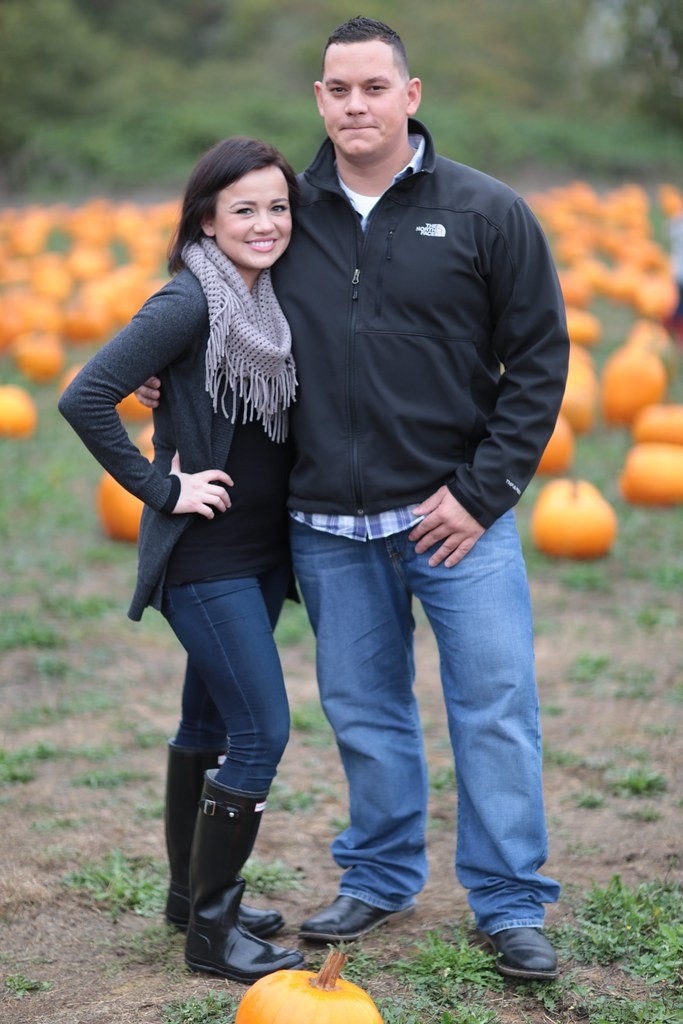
[0,177,682,560]
[234,946,385,1024]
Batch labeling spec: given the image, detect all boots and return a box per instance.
[166,738,285,936]
[186,770,304,984]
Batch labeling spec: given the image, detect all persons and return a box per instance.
[61,138,306,983]
[131,18,570,978]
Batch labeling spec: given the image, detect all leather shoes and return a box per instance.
[298,895,415,940]
[477,922,559,977]
